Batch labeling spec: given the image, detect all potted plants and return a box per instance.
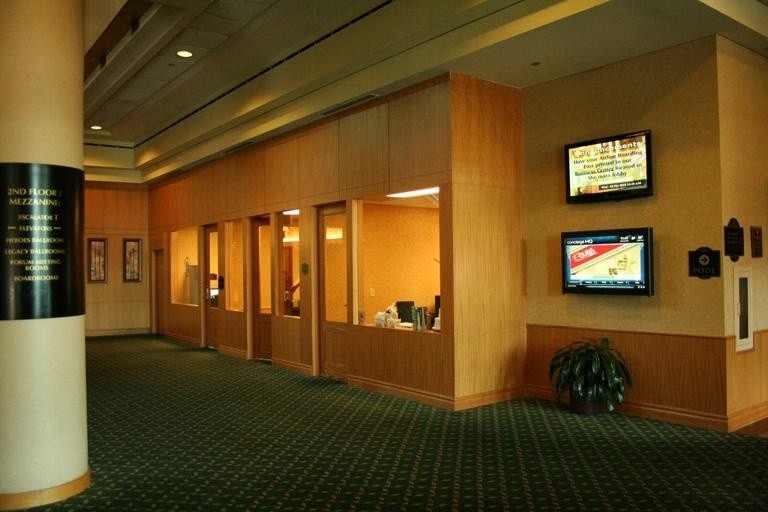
[546,335,633,417]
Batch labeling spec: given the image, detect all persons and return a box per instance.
[287,281,300,296]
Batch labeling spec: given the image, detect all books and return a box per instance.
[412,306,429,329]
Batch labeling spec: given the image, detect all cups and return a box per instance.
[426,313,433,330]
[411,306,427,331]
[434,317,440,328]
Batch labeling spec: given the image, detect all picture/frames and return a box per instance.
[86,236,108,285]
[122,237,143,283]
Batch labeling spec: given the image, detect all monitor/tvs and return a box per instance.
[395,301,414,322]
[210,288,219,299]
[561,227,655,298]
[434,295,441,317]
[564,130,653,205]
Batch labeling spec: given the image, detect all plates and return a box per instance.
[432,327,441,331]
[400,322,413,328]
